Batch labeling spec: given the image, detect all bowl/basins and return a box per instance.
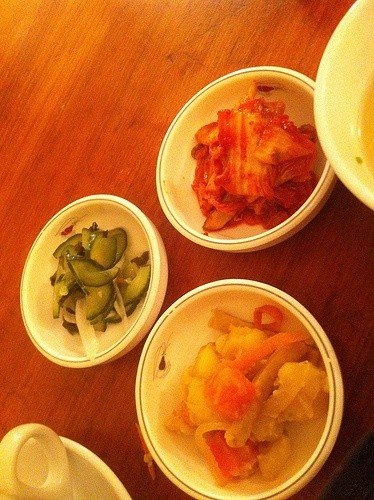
[155,67,337,253]
[135,278,345,500]
[20,195,168,369]
[313,0,374,211]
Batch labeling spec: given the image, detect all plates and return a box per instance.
[0,433,132,500]
